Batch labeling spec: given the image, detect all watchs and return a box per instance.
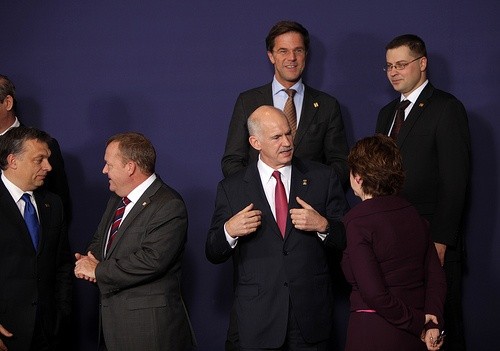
[319,225,329,234]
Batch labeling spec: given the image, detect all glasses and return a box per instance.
[383,56,423,72]
[272,48,305,55]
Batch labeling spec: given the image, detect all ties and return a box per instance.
[390,99,413,143]
[106,196,130,258]
[283,89,297,139]
[272,171,288,239]
[22,193,40,251]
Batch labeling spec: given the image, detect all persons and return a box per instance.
[74,132,197,351]
[374,34,474,267]
[341,134,450,351]
[207,105,350,351]
[0,126,69,350]
[221,20,349,177]
[0,76,65,243]
